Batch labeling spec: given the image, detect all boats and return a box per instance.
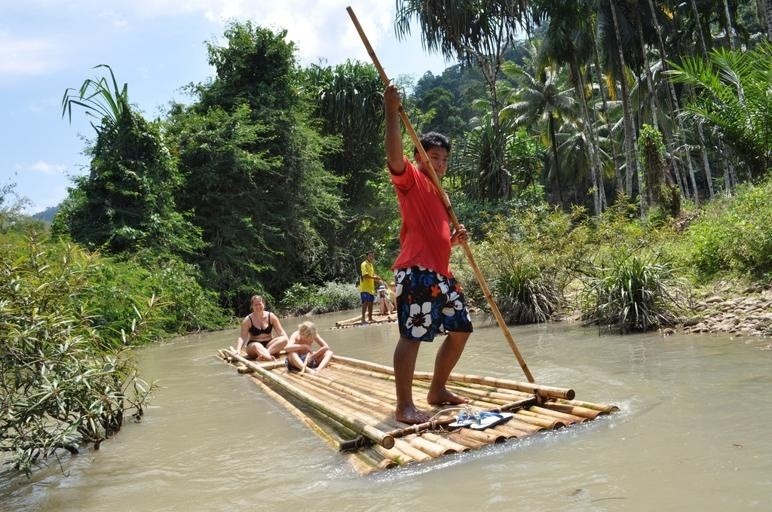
[330,309,397,330]
[216,346,619,475]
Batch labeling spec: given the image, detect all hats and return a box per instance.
[377,286,386,291]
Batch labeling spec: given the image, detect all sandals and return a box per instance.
[448,410,513,430]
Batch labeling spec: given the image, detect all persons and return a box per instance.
[374,275,398,316]
[360,252,382,322]
[234,295,288,362]
[283,321,334,373]
[383,84,473,425]
